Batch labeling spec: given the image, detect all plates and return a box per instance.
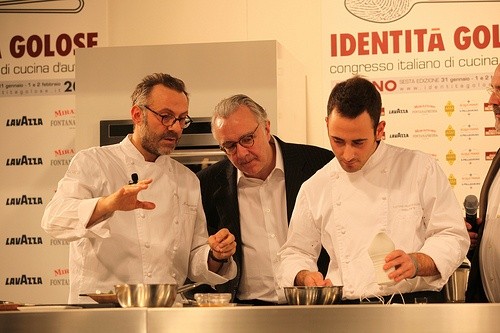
[0,304,20,310]
[88,293,117,303]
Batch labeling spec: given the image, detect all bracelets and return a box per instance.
[411,255,418,279]
[210,249,230,262]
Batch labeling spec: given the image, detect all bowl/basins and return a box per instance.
[283,286,344,305]
[194,292,232,306]
[113,284,180,308]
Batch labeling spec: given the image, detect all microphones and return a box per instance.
[129,172,139,184]
[463,194,480,234]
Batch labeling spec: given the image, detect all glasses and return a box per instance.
[145,106,193,129]
[220,124,260,156]
[487,88,500,99]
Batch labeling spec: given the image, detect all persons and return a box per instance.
[464,65,500,304]
[41,72,236,304]
[193,93,334,307]
[275,76,471,304]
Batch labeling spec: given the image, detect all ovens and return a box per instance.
[100,117,228,175]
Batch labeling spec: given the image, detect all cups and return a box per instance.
[445,262,471,303]
